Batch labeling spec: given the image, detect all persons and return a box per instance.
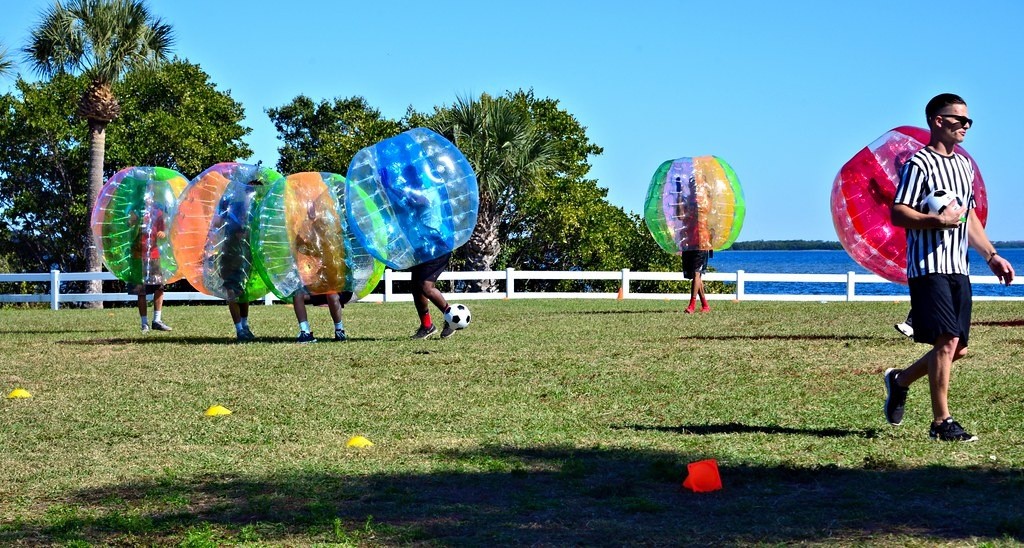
[883,93,1016,445]
[291,200,347,342]
[378,166,458,338]
[674,175,715,313]
[214,180,267,341]
[128,184,173,335]
[869,151,926,338]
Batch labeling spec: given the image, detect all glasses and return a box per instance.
[939,115,973,128]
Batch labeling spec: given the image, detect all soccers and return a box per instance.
[920,189,962,216]
[444,303,471,330]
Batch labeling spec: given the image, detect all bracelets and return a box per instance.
[985,253,997,264]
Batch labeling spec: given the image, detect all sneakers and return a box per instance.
[142,327,149,332]
[894,322,914,338]
[238,334,249,340]
[296,333,317,343]
[247,332,254,341]
[335,330,345,341]
[440,324,457,339]
[409,324,438,341]
[700,306,710,313]
[685,307,695,313]
[884,368,908,426]
[929,418,978,443]
[152,321,172,331]
